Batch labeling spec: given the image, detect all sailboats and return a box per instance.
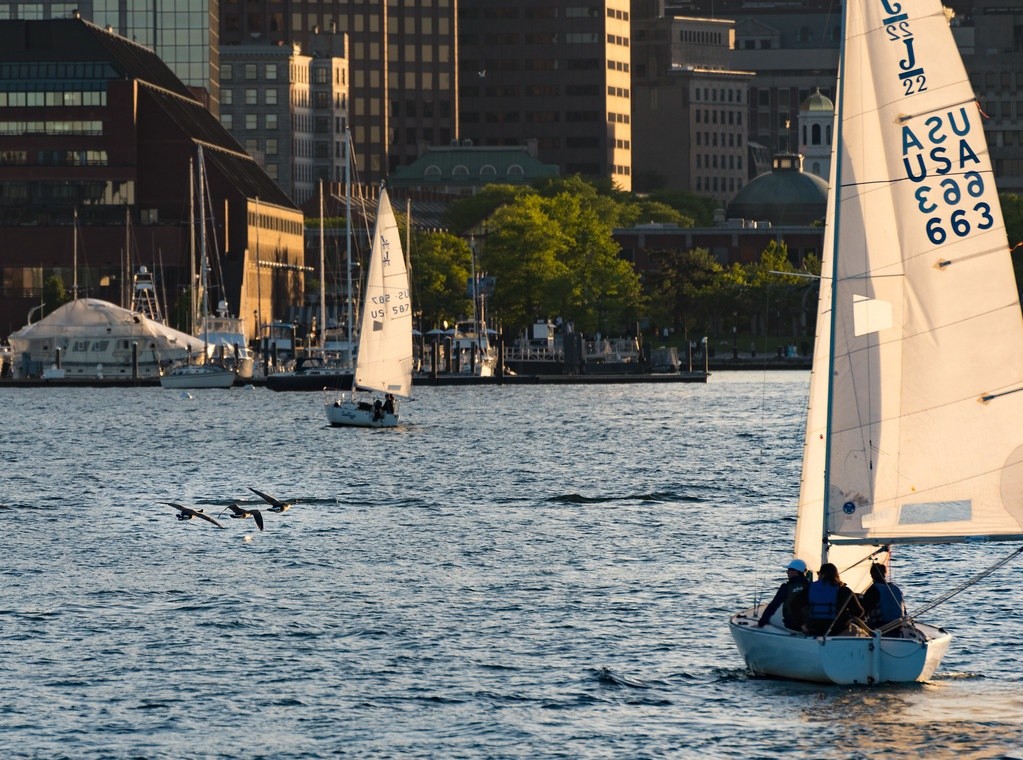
[447,231,500,377]
[322,179,414,428]
[321,125,374,360]
[726,0,1023,687]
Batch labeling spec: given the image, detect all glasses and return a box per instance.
[816,572,821,575]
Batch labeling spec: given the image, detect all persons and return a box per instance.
[750,559,865,637]
[751,342,756,357]
[860,563,907,638]
[374,393,396,414]
[787,342,798,357]
[801,339,808,356]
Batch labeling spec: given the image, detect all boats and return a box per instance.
[262,336,354,391]
[7,206,256,381]
[646,344,682,374]
[504,316,645,374]
[157,342,236,389]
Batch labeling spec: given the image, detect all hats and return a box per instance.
[783,559,807,573]
